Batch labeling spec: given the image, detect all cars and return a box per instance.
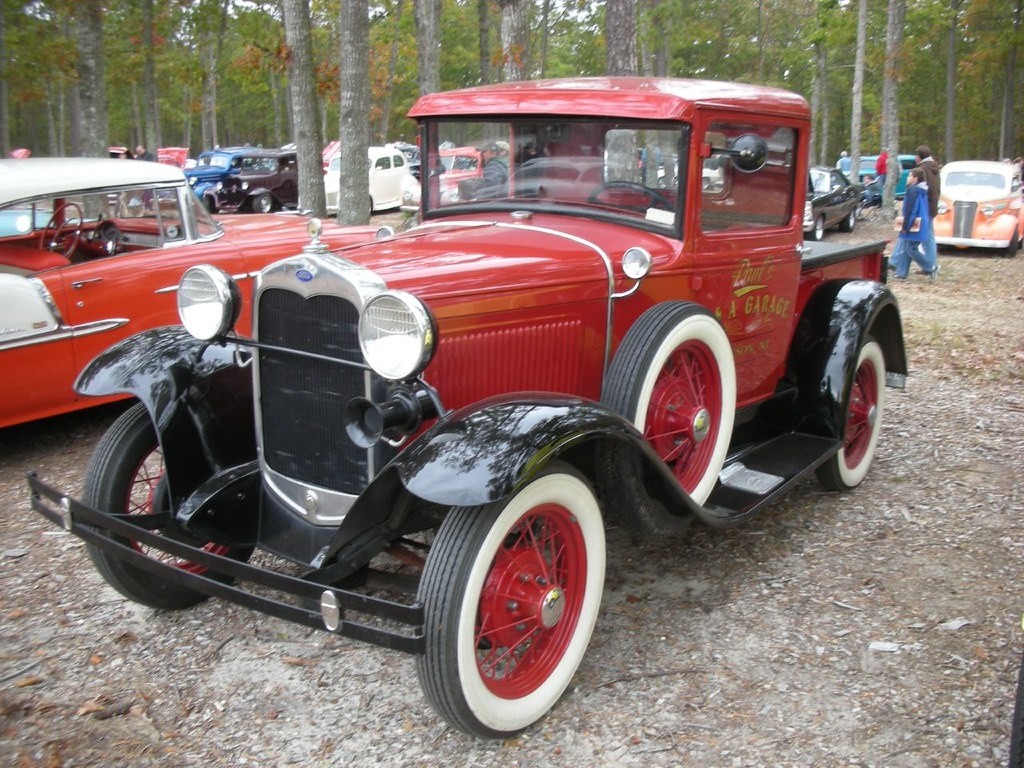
[798,164,869,240]
[399,145,505,216]
[0,156,398,423]
[394,145,424,180]
[838,155,924,203]
[636,147,725,192]
[323,146,418,217]
[894,159,1024,260]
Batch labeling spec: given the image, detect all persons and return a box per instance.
[890,167,941,283]
[887,145,941,275]
[136,145,159,212]
[836,147,903,217]
[459,141,510,199]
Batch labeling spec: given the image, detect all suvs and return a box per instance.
[27,75,908,735]
[175,142,300,215]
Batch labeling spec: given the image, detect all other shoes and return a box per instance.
[887,263,896,271]
[916,270,929,275]
[887,275,907,281]
[931,264,939,281]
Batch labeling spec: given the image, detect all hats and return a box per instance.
[475,143,500,152]
[840,151,848,157]
[496,141,509,151]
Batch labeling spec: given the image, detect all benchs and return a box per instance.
[0,244,71,272]
